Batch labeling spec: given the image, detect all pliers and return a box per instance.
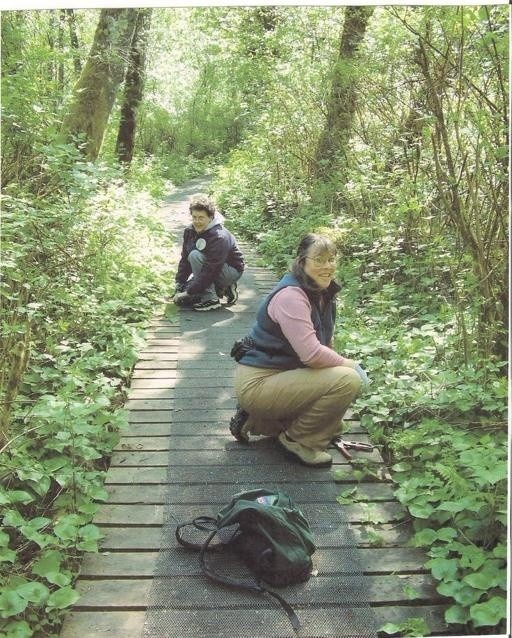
[332,436,372,459]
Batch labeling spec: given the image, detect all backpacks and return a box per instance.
[175,489,319,593]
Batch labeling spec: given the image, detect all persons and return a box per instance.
[228,233,372,467]
[171,194,245,312]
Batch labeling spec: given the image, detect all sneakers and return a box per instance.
[277,432,333,467]
[226,280,239,305]
[193,297,221,312]
[230,404,250,443]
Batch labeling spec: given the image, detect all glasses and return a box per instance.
[305,255,336,267]
